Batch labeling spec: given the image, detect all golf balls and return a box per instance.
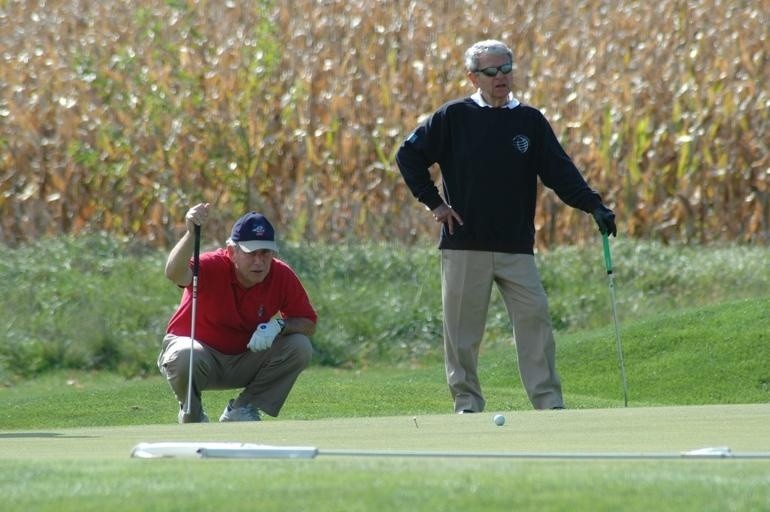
[494,416,505,426]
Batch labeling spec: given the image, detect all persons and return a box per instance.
[396,39,616,414]
[156,204,317,422]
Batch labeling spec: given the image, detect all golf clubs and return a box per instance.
[179,225,200,422]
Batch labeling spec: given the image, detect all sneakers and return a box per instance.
[178,405,209,423]
[219,398,261,421]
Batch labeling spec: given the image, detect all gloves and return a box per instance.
[247,319,282,353]
[591,204,616,237]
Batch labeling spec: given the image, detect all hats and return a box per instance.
[231,211,279,253]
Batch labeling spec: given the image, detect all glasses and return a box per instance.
[474,62,512,77]
